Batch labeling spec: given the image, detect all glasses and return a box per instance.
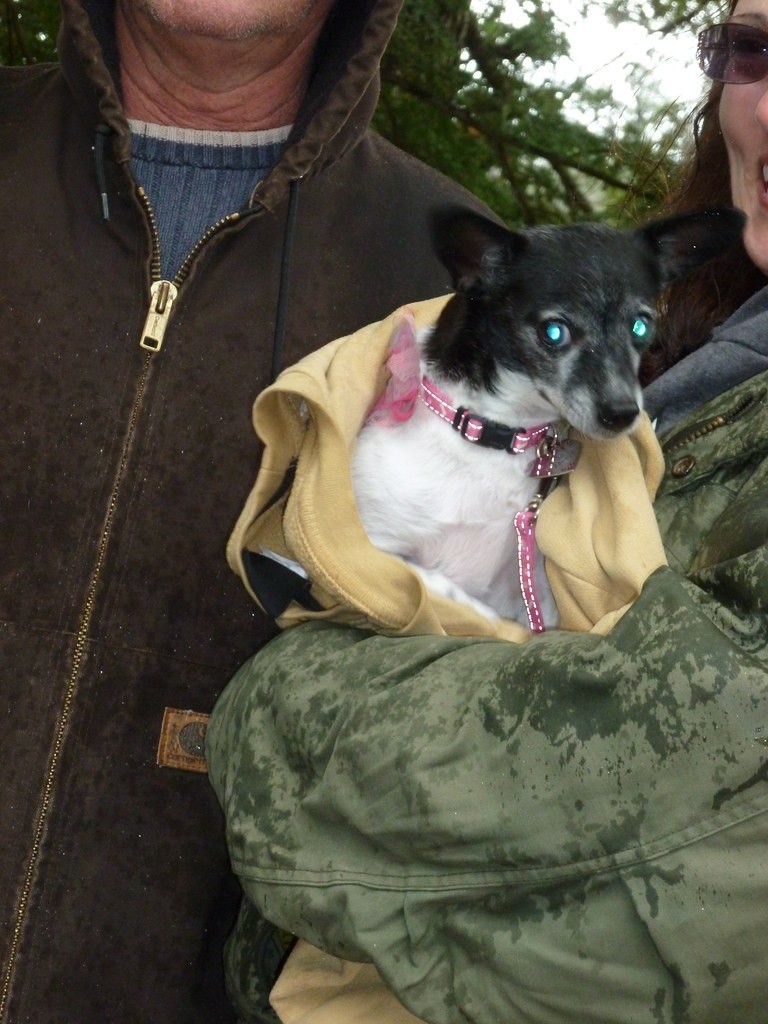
[694,21,768,84]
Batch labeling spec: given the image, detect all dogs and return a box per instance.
[217,200,749,655]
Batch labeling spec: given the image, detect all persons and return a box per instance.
[2,2,508,1024]
[206,1,766,1024]
